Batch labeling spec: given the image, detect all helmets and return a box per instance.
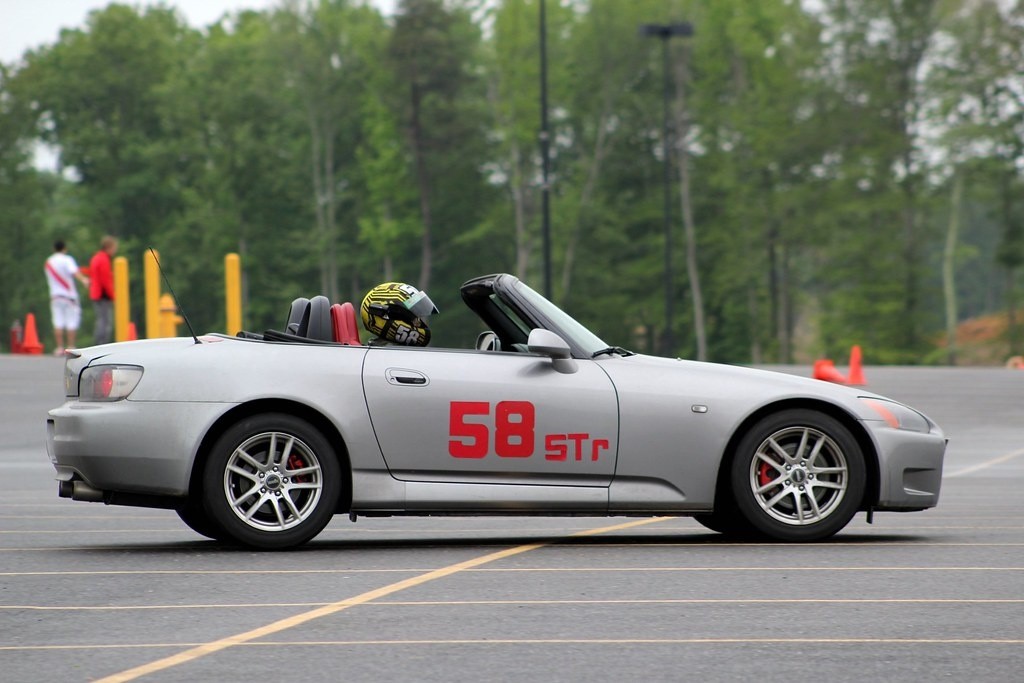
[359,282,440,347]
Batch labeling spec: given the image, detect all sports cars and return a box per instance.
[42,270,953,553]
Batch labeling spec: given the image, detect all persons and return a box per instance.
[44,240,88,353]
[89,235,116,345]
[361,283,440,348]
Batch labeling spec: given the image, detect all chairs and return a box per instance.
[333,305,361,345]
[343,302,361,343]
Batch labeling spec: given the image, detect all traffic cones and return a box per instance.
[129,320,139,340]
[813,359,847,384]
[845,342,870,386]
[19,311,46,353]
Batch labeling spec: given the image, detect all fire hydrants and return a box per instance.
[10,319,22,354]
[160,289,184,339]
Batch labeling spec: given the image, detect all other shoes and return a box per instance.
[53,348,64,357]
